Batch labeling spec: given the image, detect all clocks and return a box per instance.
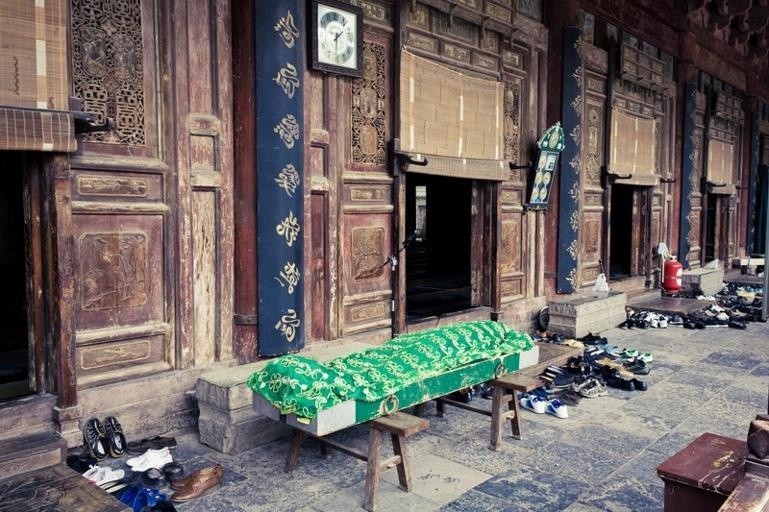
[307,0,365,80]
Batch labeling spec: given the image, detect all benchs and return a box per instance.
[283,410,430,511]
[429,371,542,453]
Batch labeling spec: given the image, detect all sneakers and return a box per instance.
[619,283,764,330]
[66,415,223,512]
[451,330,654,419]
